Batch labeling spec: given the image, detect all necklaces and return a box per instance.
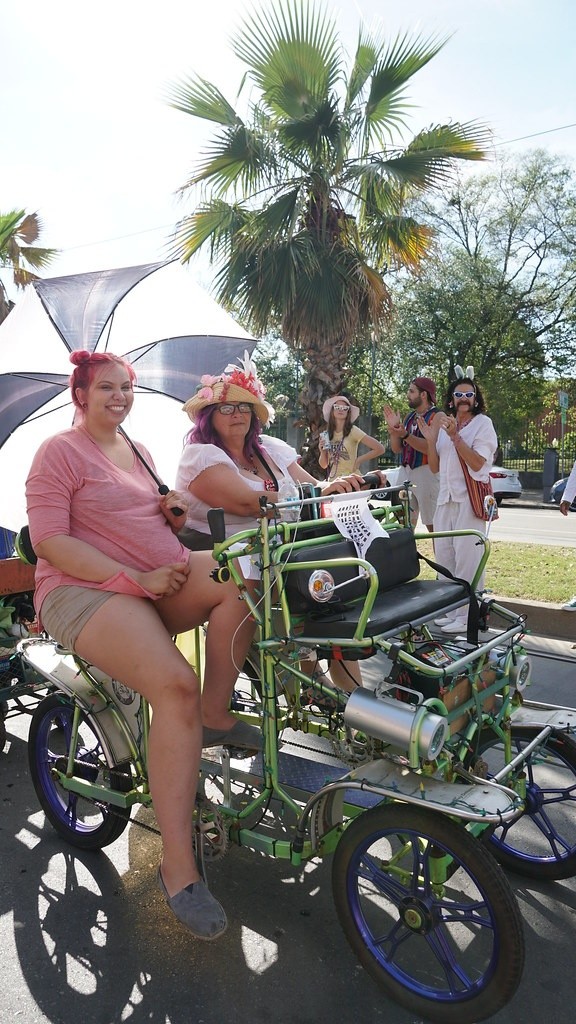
[326,430,344,479]
[402,404,434,446]
[456,414,473,433]
[221,445,276,491]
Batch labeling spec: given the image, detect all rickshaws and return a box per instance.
[0,475,576,1024]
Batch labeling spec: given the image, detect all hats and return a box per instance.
[412,378,437,404]
[323,396,360,425]
[182,349,275,429]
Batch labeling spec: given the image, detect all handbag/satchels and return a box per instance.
[456,453,499,521]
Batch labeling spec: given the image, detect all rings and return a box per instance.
[447,425,450,429]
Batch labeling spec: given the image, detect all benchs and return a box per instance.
[281,524,481,655]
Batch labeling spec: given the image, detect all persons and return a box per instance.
[417,377,499,633]
[560,457,576,613]
[23,350,259,941]
[175,374,387,709]
[384,377,448,581]
[319,395,385,482]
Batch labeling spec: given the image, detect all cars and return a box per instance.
[551,476,576,513]
[373,465,522,506]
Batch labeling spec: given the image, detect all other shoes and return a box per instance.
[158,864,227,940]
[202,720,284,751]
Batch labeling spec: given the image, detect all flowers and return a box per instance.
[189,354,279,423]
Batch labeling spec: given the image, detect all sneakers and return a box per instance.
[441,621,466,633]
[434,616,455,626]
[562,599,576,611]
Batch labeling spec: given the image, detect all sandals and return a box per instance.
[300,671,352,712]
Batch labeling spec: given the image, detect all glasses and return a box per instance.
[452,392,474,399]
[212,402,254,415]
[333,405,351,412]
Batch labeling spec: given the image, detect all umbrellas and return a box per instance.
[0,257,262,535]
